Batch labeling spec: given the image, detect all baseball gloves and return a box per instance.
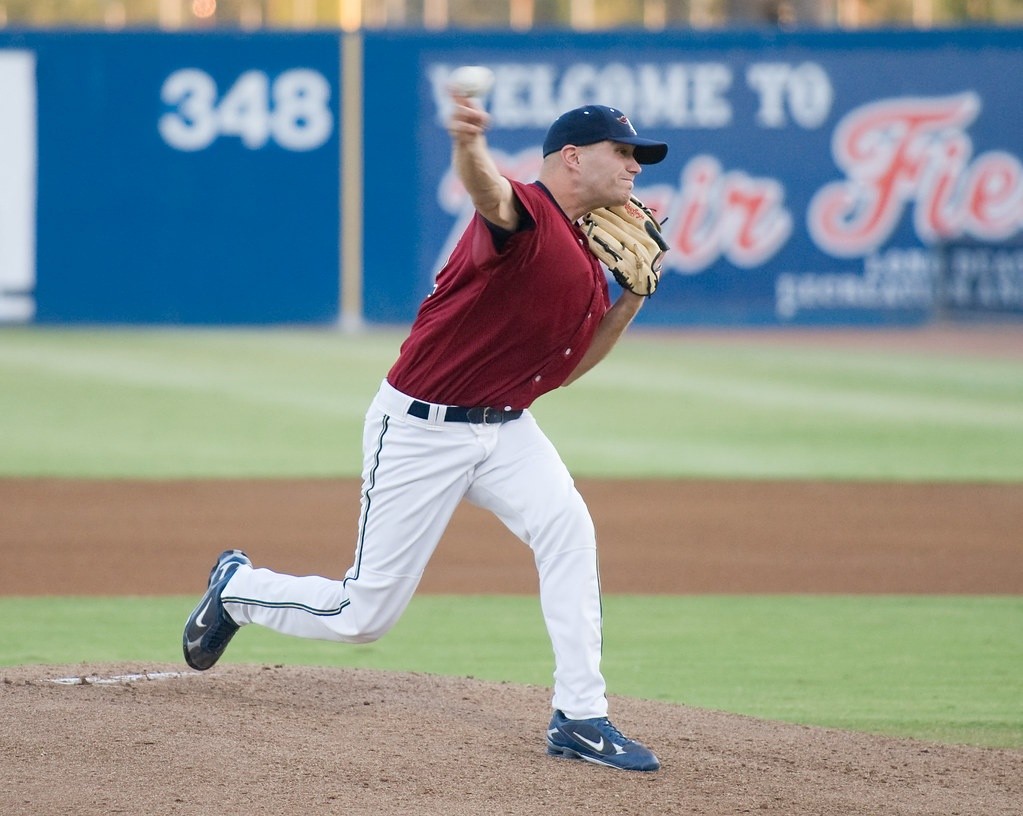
[577,191,671,296]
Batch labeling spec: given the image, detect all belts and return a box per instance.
[408,400,524,427]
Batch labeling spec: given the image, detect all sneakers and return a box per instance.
[545,709,660,771]
[182,548,253,671]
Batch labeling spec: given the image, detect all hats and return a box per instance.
[543,104,669,166]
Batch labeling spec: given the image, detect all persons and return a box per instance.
[182,97,669,772]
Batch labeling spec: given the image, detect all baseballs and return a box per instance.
[446,64,495,98]
